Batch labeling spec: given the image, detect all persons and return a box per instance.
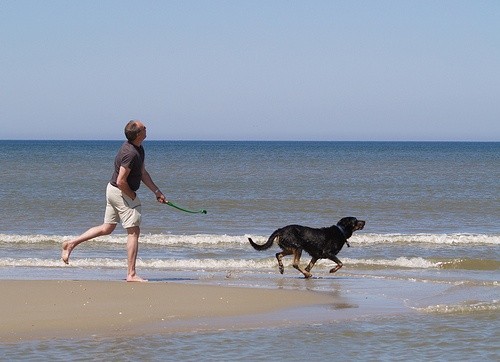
[61,120,169,282]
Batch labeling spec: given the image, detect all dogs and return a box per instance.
[248,216,365,278]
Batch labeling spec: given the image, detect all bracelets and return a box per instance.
[154,189,160,195]
